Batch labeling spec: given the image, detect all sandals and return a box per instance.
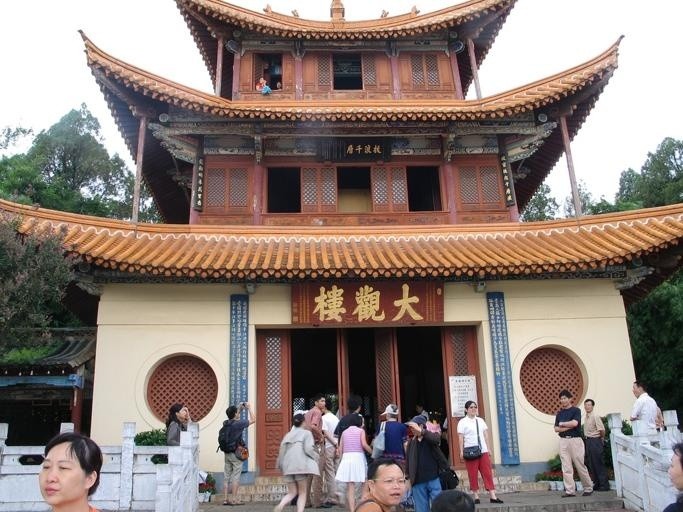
[223,499,245,505]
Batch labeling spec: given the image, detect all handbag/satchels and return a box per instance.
[235,446,249,461]
[438,469,459,490]
[463,446,481,460]
[371,430,385,459]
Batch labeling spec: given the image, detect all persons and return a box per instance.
[167,403,192,445]
[554,390,593,496]
[39,432,103,512]
[223,402,256,505]
[256,76,267,91]
[629,381,660,450]
[583,399,609,491]
[273,392,503,512]
[663,443,683,512]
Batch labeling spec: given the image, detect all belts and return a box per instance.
[561,436,579,438]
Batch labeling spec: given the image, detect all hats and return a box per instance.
[404,416,426,426]
[380,404,399,416]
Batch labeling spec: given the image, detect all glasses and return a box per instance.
[469,406,478,409]
[372,478,406,487]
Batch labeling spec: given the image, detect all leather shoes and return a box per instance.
[317,504,331,508]
[583,491,593,496]
[474,499,480,504]
[562,493,575,497]
[490,497,503,503]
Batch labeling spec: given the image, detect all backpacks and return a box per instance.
[218,420,242,453]
